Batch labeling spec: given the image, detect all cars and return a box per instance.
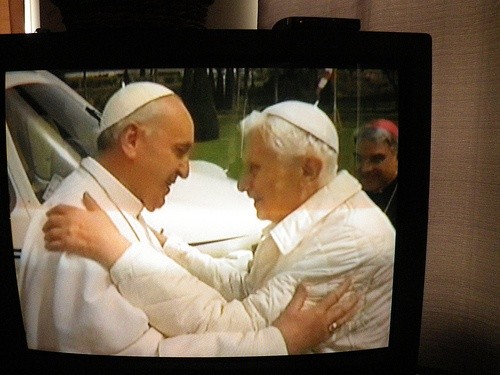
[5,70,273,259]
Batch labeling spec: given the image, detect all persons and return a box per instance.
[42,101,395,354]
[350,118,399,347]
[22,83,366,357]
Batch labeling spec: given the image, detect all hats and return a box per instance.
[374,120,398,138]
[99,80,175,133]
[262,100,339,153]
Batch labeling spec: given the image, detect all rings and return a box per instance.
[328,322,339,334]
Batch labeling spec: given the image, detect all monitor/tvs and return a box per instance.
[0,27,432,375]
